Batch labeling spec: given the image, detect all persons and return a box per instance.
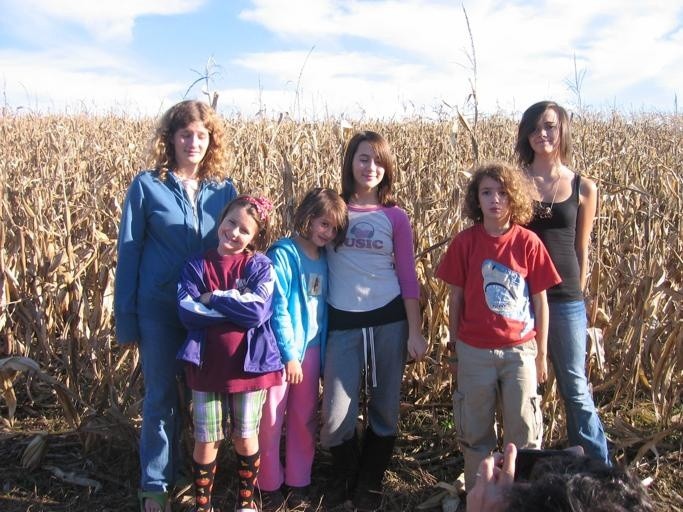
[464,442,636,511]
[319,132,428,512]
[113,100,237,511]
[175,194,274,512]
[257,189,349,507]
[512,100,613,468]
[433,164,550,493]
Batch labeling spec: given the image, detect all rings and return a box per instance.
[472,473,481,478]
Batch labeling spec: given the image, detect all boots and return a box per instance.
[322,437,352,506]
[353,430,396,509]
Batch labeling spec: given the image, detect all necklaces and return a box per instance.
[524,166,566,220]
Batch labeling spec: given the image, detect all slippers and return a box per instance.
[137,488,171,512]
